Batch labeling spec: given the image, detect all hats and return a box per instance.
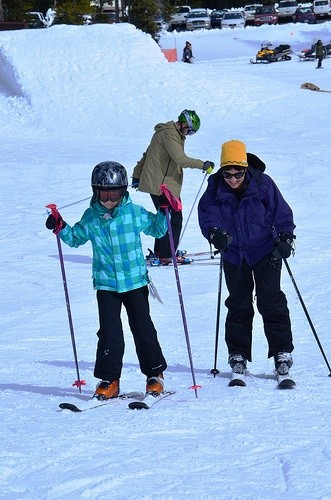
[220,139,248,167]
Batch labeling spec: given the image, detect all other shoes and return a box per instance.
[155,251,185,266]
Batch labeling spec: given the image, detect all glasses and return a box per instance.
[222,172,245,179]
[187,125,196,136]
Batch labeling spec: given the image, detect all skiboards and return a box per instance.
[58,390,177,413]
[143,246,223,267]
[226,349,296,388]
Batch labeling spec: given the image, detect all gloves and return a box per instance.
[157,192,174,215]
[207,227,234,250]
[202,161,214,174]
[46,214,63,230]
[272,231,296,259]
[131,178,139,188]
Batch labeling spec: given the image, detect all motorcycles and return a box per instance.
[250,40,292,64]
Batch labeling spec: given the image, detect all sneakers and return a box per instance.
[228,353,247,373]
[274,351,293,374]
[146,373,164,396]
[92,378,119,400]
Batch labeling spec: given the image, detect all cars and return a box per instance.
[221,10,246,29]
[293,7,317,24]
[253,6,278,24]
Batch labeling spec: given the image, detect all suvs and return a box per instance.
[311,0,330,18]
[244,4,263,21]
[169,5,191,26]
[184,8,211,31]
[276,0,301,19]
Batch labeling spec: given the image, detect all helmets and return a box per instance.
[178,109,200,131]
[92,161,128,197]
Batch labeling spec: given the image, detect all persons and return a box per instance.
[130,109,215,265]
[45,161,172,401]
[197,140,297,375]
[315,40,325,69]
[181,41,195,64]
